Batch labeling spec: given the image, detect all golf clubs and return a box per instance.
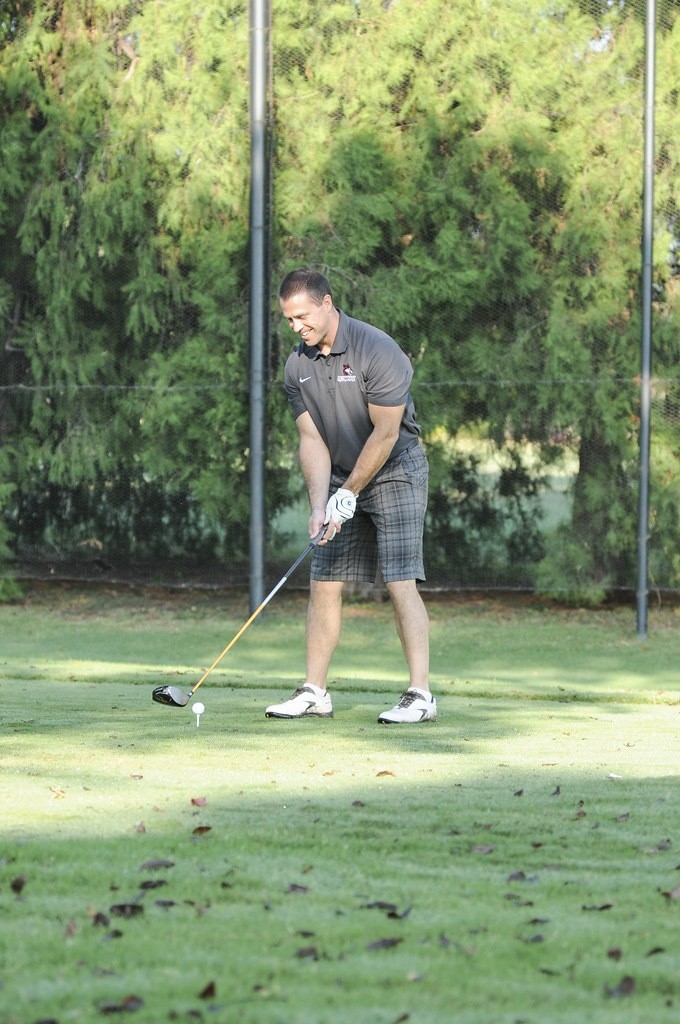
[152,522,331,707]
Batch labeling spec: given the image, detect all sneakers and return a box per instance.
[264,687,333,719]
[379,690,437,722]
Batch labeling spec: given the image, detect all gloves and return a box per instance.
[322,488,356,541]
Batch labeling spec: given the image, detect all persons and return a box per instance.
[264,268,438,723]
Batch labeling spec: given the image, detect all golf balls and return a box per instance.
[192,702,205,715]
